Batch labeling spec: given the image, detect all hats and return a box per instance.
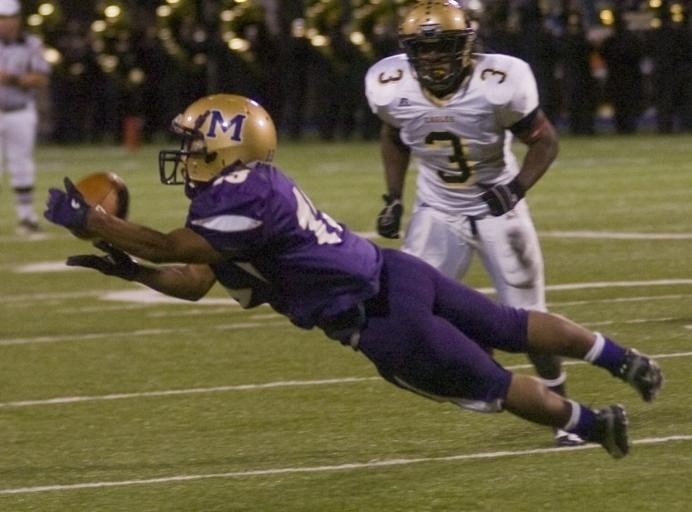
[0,0,21,16]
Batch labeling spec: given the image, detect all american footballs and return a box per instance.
[69,172,129,239]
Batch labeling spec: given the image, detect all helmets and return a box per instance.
[159,94,278,199]
[398,0,475,91]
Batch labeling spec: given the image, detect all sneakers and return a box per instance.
[613,347,662,402]
[594,403,629,459]
[555,429,585,447]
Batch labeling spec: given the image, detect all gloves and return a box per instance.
[475,181,525,216]
[378,194,402,238]
[67,240,138,281]
[44,177,90,228]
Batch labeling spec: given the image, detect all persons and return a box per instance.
[42,92,665,464]
[362,0,570,404]
[2,0,54,245]
[21,0,692,155]
